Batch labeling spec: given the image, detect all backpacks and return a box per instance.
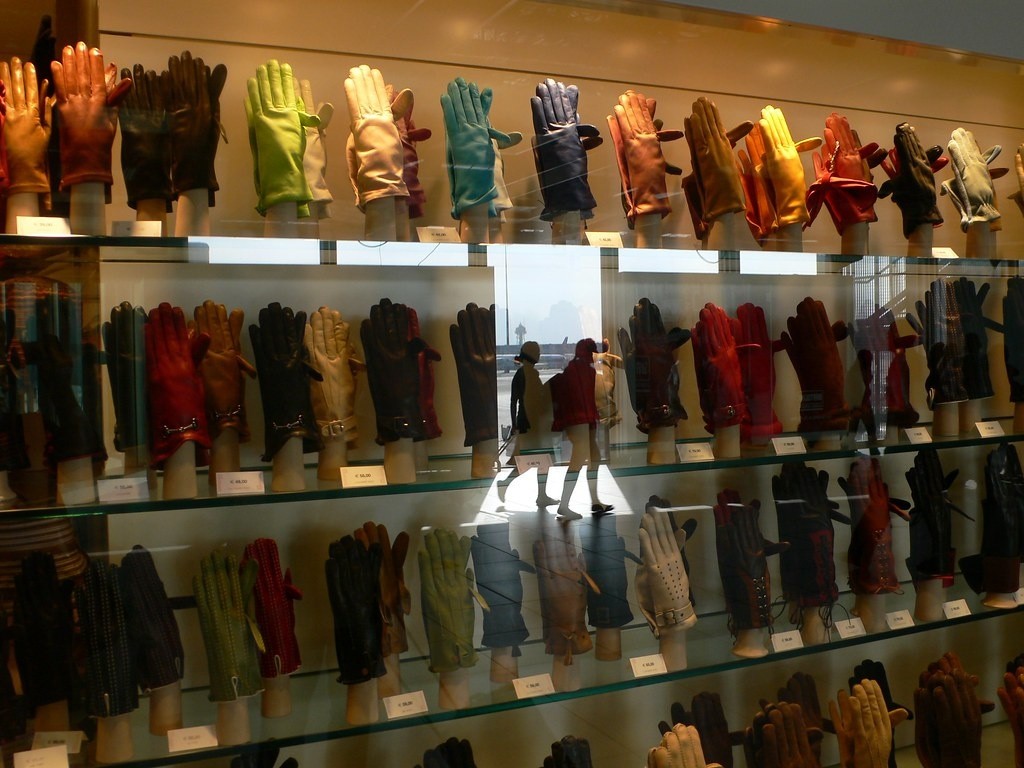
[543,360,581,432]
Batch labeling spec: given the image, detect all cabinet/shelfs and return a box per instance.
[0,234,1023,768]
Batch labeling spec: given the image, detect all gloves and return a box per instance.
[0,442,1024,718]
[0,272,1024,481]
[1,42,1023,240]
[231,651,1024,768]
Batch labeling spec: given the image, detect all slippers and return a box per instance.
[555,512,582,520]
[592,504,614,516]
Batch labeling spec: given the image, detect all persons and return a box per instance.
[497,339,614,521]
